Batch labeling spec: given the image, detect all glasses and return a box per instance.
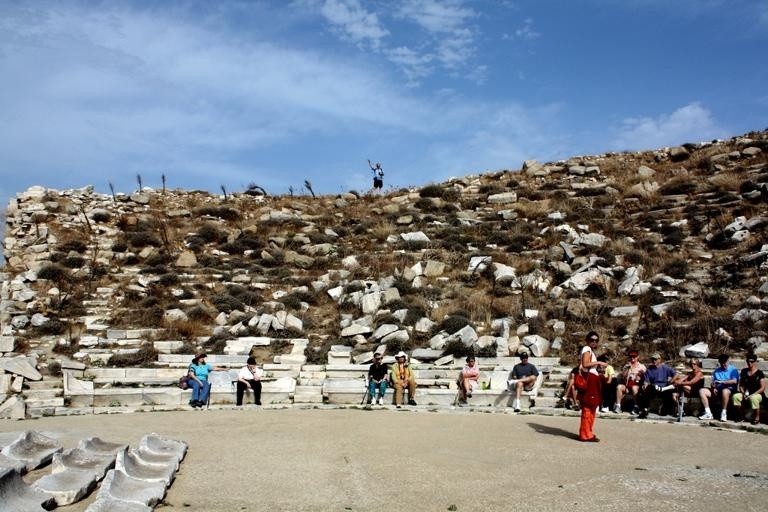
[588,337,598,343]
[377,357,383,360]
[745,359,756,364]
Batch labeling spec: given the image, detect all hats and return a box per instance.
[520,351,528,359]
[629,350,639,356]
[192,348,206,363]
[247,356,257,365]
[651,353,660,359]
[395,351,408,361]
[718,353,729,364]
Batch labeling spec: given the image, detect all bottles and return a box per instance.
[745,389,749,397]
[482,381,487,389]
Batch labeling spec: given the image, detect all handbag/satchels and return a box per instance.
[180,376,189,389]
[573,372,587,389]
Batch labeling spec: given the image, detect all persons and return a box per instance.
[506,351,540,412]
[389,349,418,409]
[556,353,763,426]
[456,352,481,404]
[365,352,389,405]
[367,158,384,192]
[235,356,262,406]
[578,330,609,442]
[186,349,214,408]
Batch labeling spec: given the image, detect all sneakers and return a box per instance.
[466,390,472,399]
[752,419,758,424]
[512,405,520,412]
[581,434,600,442]
[600,402,686,417]
[507,378,515,386]
[189,400,203,407]
[720,412,727,422]
[370,399,418,408]
[699,413,713,420]
[555,395,580,411]
